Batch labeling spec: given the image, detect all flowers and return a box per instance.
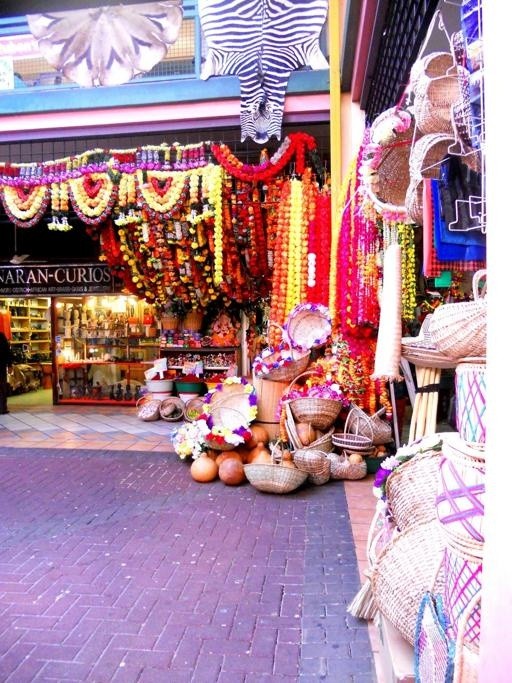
[371,434,444,504]
[171,375,259,460]
[252,303,390,418]
[355,107,412,225]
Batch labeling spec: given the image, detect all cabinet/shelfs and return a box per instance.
[8,296,51,361]
[51,295,241,407]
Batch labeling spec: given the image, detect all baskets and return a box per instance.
[401,269,486,369]
[172,375,258,460]
[136,397,183,422]
[455,363,487,451]
[241,440,309,494]
[359,51,460,226]
[347,432,487,683]
[284,372,392,493]
[253,303,335,382]
[161,318,179,330]
[171,309,394,494]
[362,269,487,683]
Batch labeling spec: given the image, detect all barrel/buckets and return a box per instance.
[174,382,204,403]
[143,380,173,401]
[204,383,223,393]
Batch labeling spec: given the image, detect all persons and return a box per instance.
[0,332,21,416]
